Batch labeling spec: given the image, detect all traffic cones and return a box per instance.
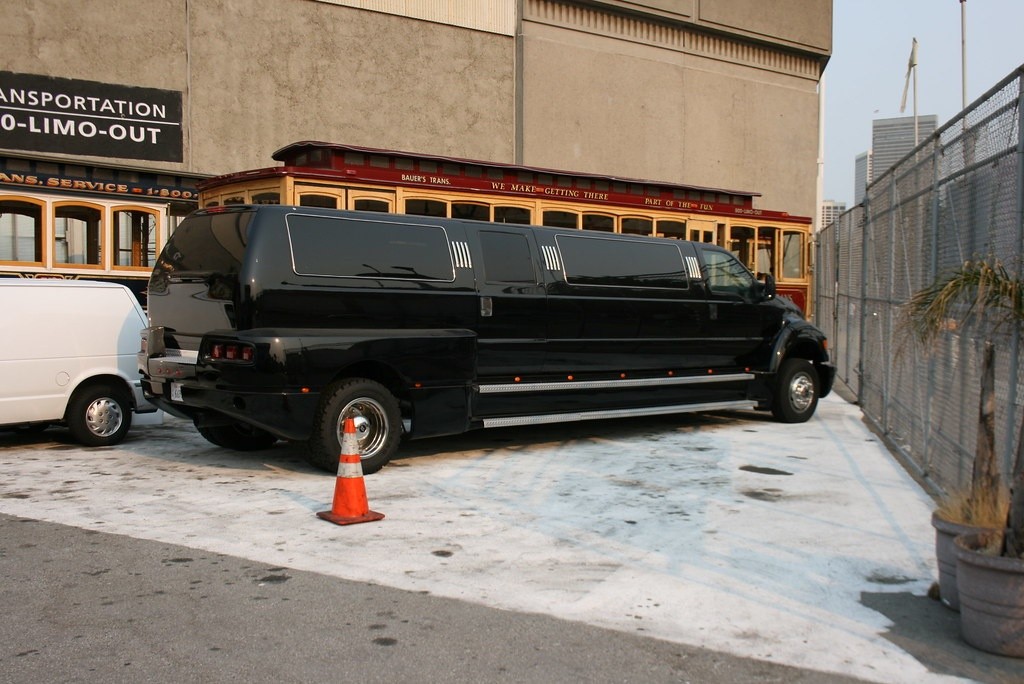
[315,419,385,527]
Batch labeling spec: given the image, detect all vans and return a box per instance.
[0,279,159,448]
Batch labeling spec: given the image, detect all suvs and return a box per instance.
[139,204,837,478]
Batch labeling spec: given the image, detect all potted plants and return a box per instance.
[894,249,1024,658]
